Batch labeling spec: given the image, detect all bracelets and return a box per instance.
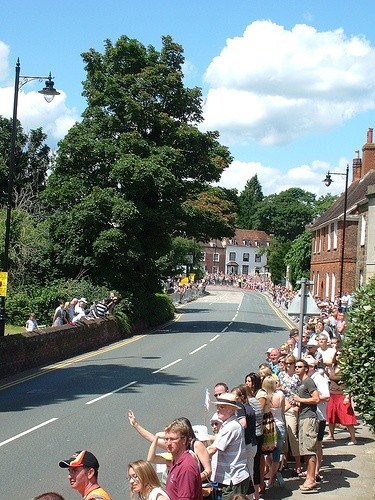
[204,471,210,477]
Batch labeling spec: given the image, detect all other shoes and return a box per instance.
[258,483,266,494]
[297,466,302,474]
[316,480,322,484]
[298,471,307,477]
[300,481,322,493]
[264,475,276,480]
[323,436,334,440]
[282,464,288,471]
[344,439,357,445]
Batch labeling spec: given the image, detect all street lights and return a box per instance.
[0,56,61,337]
[321,163,350,315]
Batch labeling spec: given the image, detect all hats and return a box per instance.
[192,425,211,441]
[212,393,242,409]
[59,450,99,470]
[304,355,316,366]
[265,348,274,353]
[210,412,223,423]
[289,329,298,336]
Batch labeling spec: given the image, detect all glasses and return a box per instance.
[286,362,294,366]
[214,393,222,397]
[294,366,306,368]
[215,405,231,410]
[278,361,283,363]
[126,474,138,482]
[163,436,186,442]
[211,423,221,427]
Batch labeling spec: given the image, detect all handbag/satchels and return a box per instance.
[201,472,217,500]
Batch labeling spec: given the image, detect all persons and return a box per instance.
[96,294,118,317]
[213,383,229,401]
[26,314,39,332]
[210,412,224,433]
[206,272,298,310]
[265,292,363,446]
[34,492,64,500]
[64,302,70,327]
[128,460,171,500]
[127,409,215,500]
[52,299,69,326]
[230,494,250,500]
[177,281,207,303]
[206,392,256,500]
[58,450,112,500]
[231,354,323,495]
[73,300,99,323]
[147,420,205,500]
[69,298,78,326]
